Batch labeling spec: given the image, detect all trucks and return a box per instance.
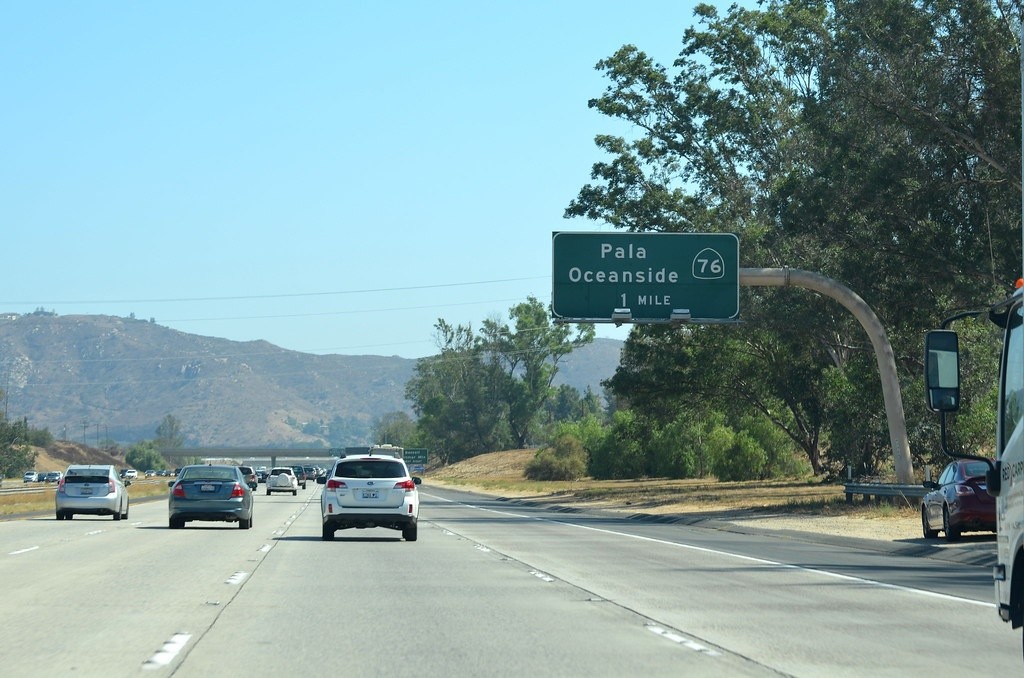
[924,280,1023,664]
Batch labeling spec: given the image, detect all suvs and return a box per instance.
[265,467,298,494]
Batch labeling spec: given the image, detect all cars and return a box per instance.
[174,468,182,477]
[156,470,166,477]
[304,466,326,482]
[921,457,997,542]
[46,471,62,483]
[239,466,258,491]
[37,472,48,482]
[119,469,129,478]
[145,470,156,478]
[316,451,423,544]
[255,470,269,483]
[23,471,38,482]
[166,463,254,529]
[54,464,132,520]
[285,465,307,490]
[125,469,138,478]
[165,470,174,477]
